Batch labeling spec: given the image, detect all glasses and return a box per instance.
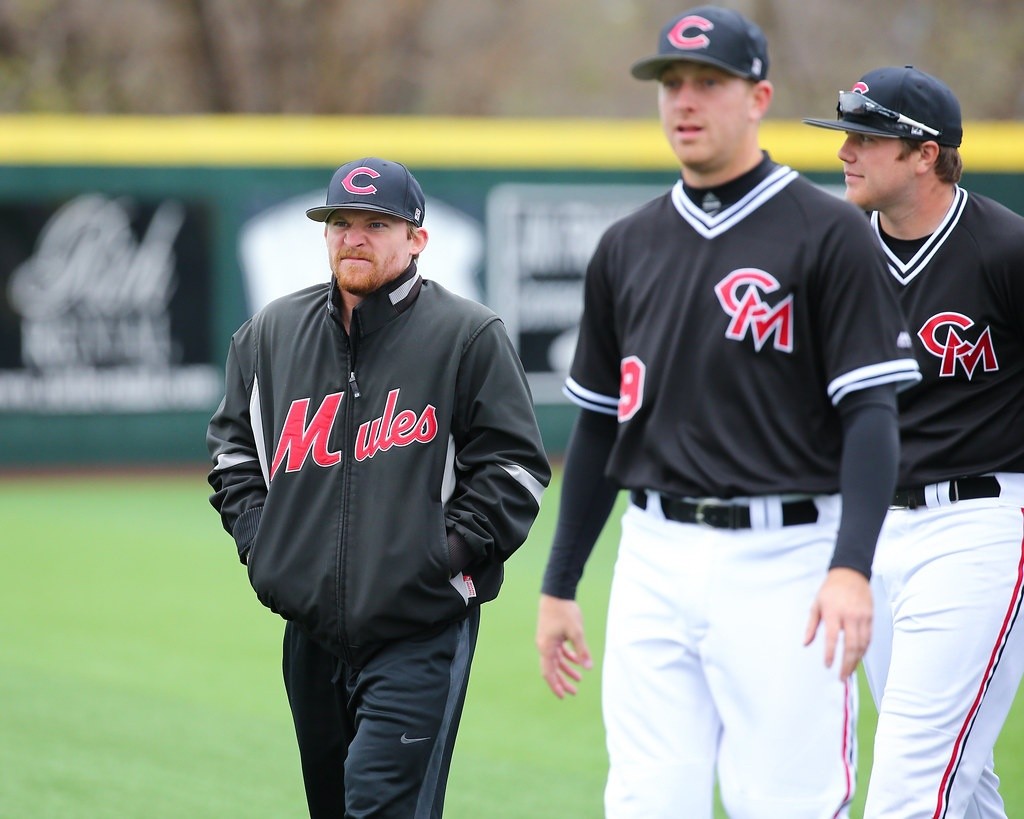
[837,90,943,138]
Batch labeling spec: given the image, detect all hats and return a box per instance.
[305,158,425,228]
[802,65,962,149]
[632,5,768,83]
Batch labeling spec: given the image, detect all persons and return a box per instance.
[799,63,1024,819]
[534,3,924,819]
[203,157,552,819]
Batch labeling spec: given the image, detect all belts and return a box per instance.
[629,486,819,530]
[889,477,1002,510]
